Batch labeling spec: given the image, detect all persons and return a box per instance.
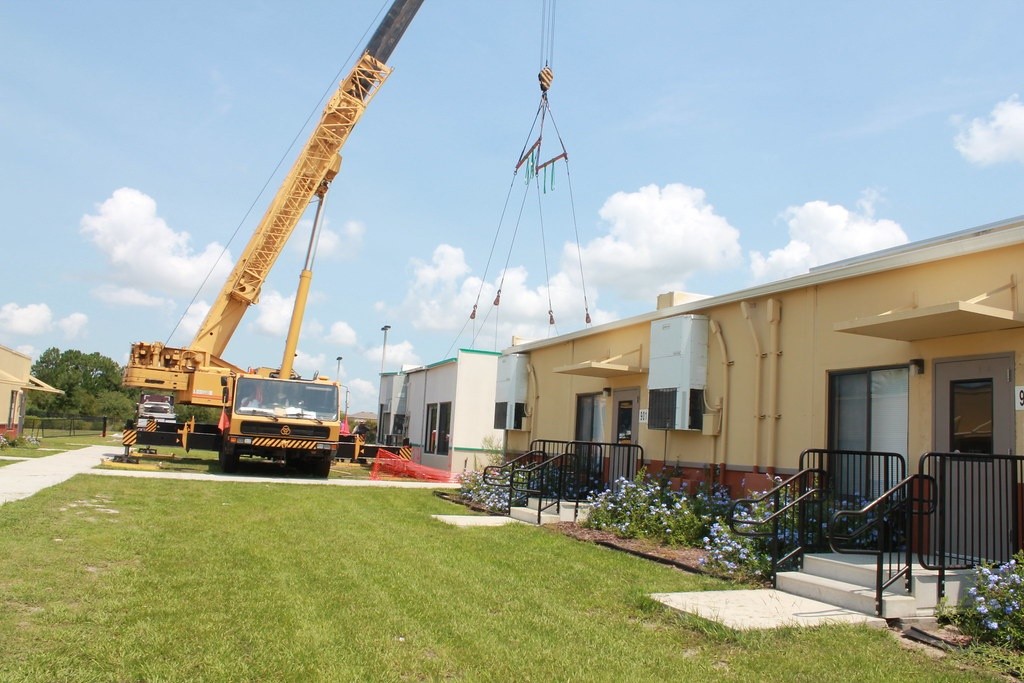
[240,389,261,408]
[352,421,370,435]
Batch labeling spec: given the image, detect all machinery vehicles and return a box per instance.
[118,1,431,478]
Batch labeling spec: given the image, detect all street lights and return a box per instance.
[336,357,342,381]
[381,325,391,373]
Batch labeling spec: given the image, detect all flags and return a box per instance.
[218,410,229,435]
